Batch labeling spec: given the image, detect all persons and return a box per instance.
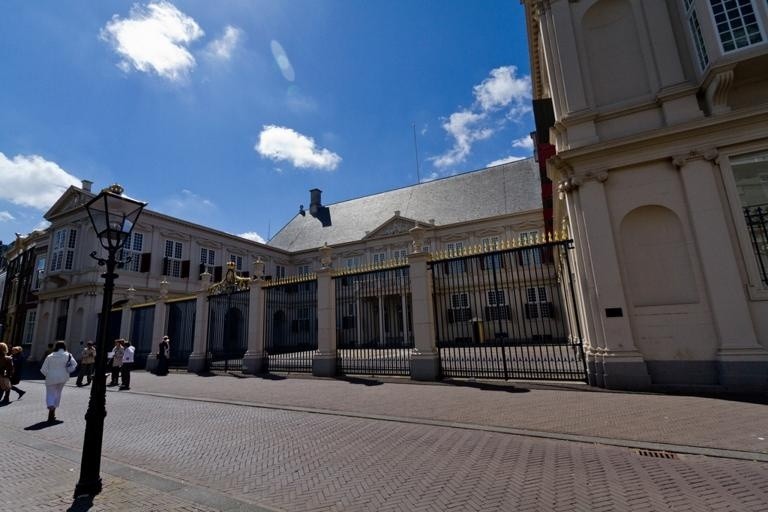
[157,336,171,376]
[41,340,96,387]
[40,341,78,423]
[107,339,135,390]
[0,342,26,405]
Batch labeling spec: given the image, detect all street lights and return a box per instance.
[81,182,148,485]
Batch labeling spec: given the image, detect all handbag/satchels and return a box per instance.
[66,353,76,374]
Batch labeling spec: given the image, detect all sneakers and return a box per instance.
[0,381,130,422]
